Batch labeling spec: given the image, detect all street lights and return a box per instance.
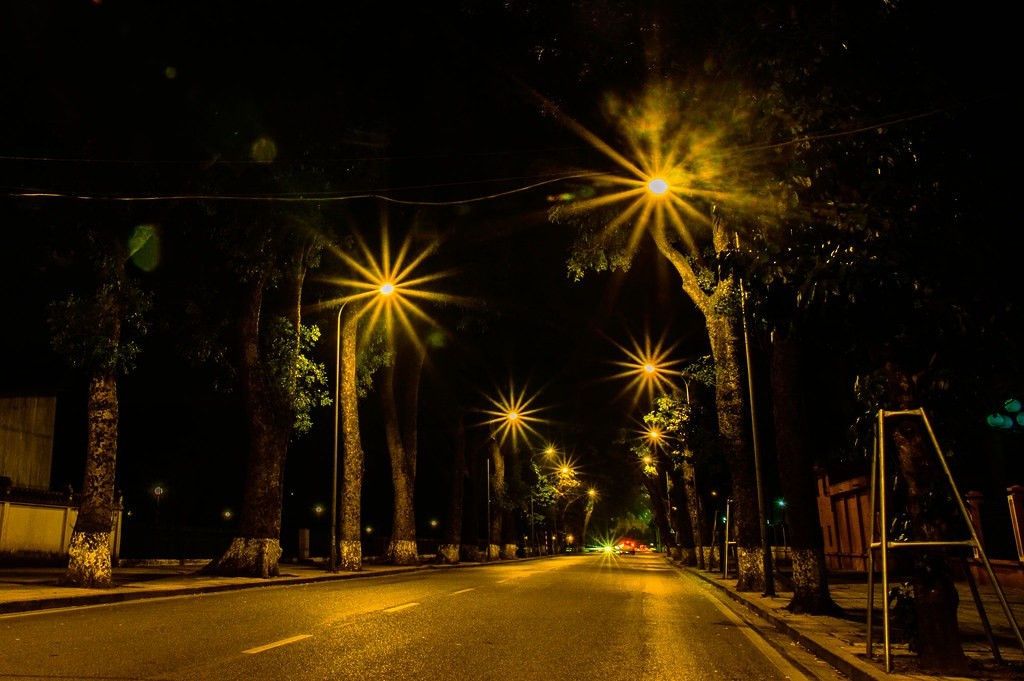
[647,174,777,598]
[329,283,395,573]
[529,447,554,559]
[643,363,705,572]
[485,411,519,562]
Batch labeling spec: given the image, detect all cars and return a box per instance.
[619,546,635,555]
